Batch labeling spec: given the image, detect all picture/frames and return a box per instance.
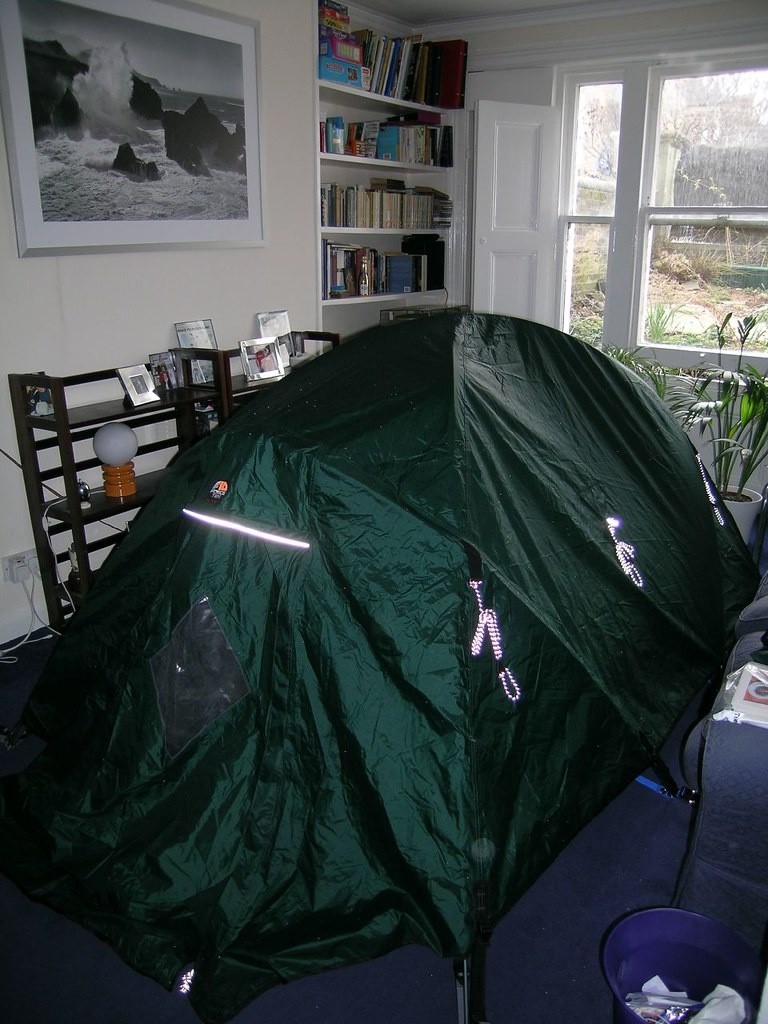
[239,335,285,384]
[114,364,161,406]
[0,0,266,258]
[256,309,295,358]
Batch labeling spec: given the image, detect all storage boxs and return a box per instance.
[388,255,416,293]
[320,37,364,64]
[387,110,442,126]
[319,0,347,15]
[320,56,370,90]
[319,25,356,40]
[399,240,447,291]
[318,6,351,34]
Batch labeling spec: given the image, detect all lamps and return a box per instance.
[93,421,142,497]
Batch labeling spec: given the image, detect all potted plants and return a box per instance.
[667,311,768,547]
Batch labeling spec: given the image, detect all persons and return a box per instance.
[159,366,172,390]
[255,345,275,372]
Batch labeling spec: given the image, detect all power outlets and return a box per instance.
[3,547,39,584]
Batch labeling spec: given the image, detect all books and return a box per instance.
[321,178,453,230]
[322,238,427,299]
[320,116,454,167]
[351,29,429,104]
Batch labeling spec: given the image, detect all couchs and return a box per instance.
[666,566,768,964]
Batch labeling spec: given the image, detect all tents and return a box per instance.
[0,313,762,1024]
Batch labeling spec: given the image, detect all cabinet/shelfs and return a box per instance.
[319,79,457,339]
[7,349,230,649]
[184,328,340,441]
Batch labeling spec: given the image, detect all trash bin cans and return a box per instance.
[604,907,761,1024]
